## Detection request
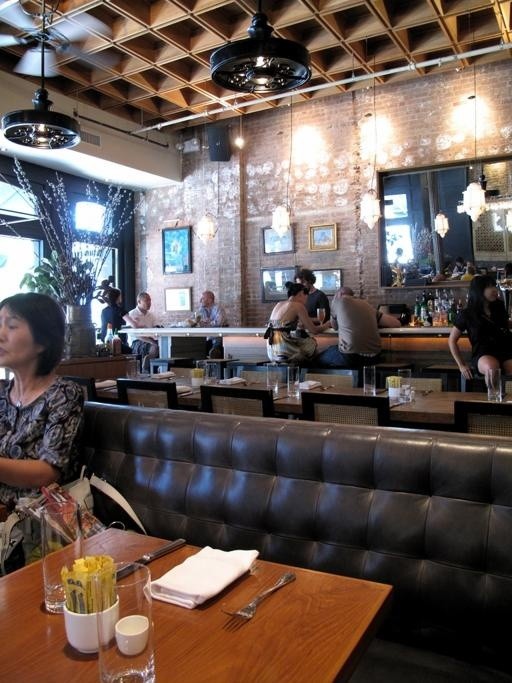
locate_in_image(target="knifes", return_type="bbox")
[116,538,185,584]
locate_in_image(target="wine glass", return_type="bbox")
[317,309,327,326]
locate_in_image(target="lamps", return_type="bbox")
[360,38,383,231]
[2,0,82,150]
[210,0,312,95]
[271,95,293,239]
[435,171,449,238]
[463,14,486,222]
[197,160,220,247]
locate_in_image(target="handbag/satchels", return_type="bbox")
[0,474,147,575]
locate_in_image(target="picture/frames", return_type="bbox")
[162,226,192,274]
[260,266,299,304]
[164,286,192,313]
[263,224,295,255]
[307,222,338,251]
[312,269,342,297]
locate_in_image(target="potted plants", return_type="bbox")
[0,156,147,357]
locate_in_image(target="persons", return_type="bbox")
[183,290,226,359]
[392,248,403,269]
[262,281,330,368]
[99,288,146,355]
[128,292,164,361]
[448,273,511,389]
[452,257,477,281]
[296,269,329,325]
[0,293,84,580]
[320,286,400,370]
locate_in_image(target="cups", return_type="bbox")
[40,501,84,613]
[388,388,400,397]
[267,363,279,397]
[115,614,149,656]
[287,366,300,397]
[93,561,156,682]
[400,369,412,402]
[497,271,506,286]
[205,364,218,384]
[63,595,120,654]
[401,384,411,395]
[363,366,376,396]
[487,368,502,402]
[191,377,203,387]
[126,360,140,380]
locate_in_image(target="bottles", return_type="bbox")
[414,288,464,327]
[96,333,104,357]
[113,328,122,353]
[105,323,114,353]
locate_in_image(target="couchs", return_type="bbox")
[67,400,512,683]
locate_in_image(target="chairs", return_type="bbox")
[378,304,411,329]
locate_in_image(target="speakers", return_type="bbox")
[205,119,235,162]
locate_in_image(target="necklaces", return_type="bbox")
[12,378,36,408]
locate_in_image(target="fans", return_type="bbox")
[0,0,121,76]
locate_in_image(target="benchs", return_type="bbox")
[97,325,229,373]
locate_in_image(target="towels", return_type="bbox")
[147,545,261,610]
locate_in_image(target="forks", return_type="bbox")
[221,571,296,619]
